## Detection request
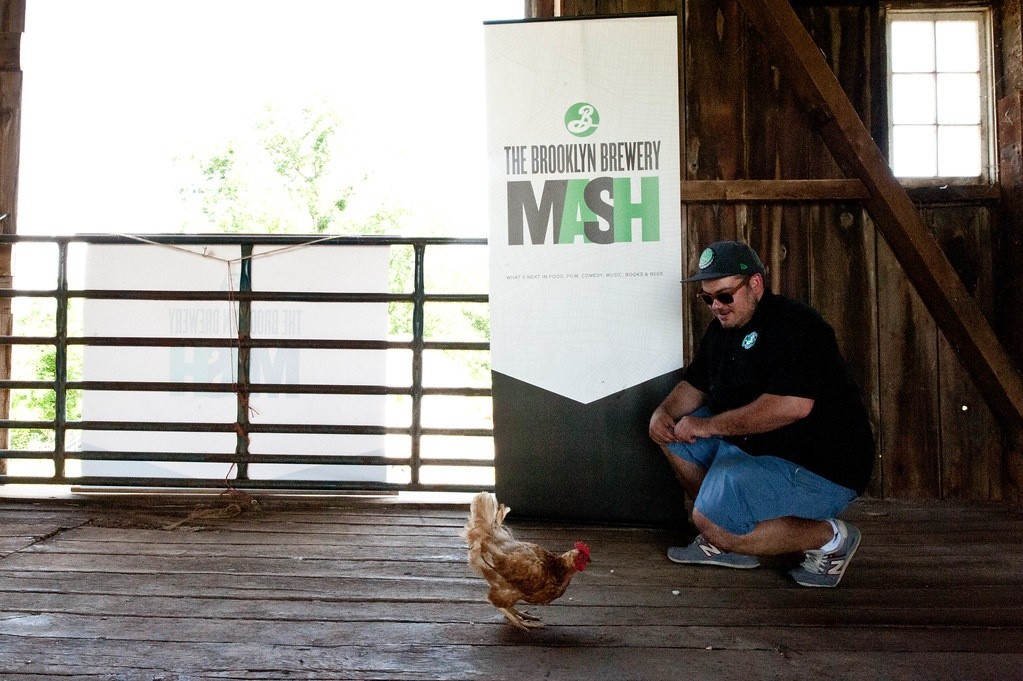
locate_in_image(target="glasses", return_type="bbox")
[697,277,752,306]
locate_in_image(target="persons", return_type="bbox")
[648,238,880,589]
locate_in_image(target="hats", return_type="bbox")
[681,241,766,282]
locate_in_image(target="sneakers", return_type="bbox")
[668,533,762,569]
[789,520,862,585]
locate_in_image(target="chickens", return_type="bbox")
[461,491,592,634]
